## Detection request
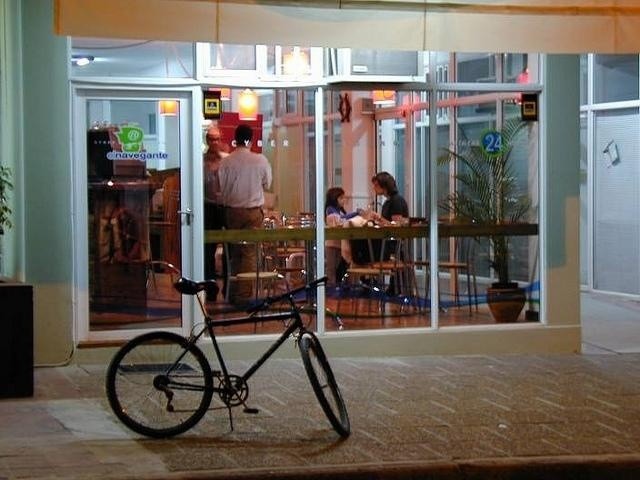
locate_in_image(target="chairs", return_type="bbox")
[222,212,478,328]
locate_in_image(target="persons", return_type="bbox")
[202,127,232,303]
[217,121,274,309]
[324,185,361,294]
[334,171,410,286]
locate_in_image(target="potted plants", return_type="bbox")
[1,163,33,398]
[438,116,532,323]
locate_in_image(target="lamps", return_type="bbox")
[160,101,176,116]
[210,87,231,100]
[372,90,396,104]
[238,89,258,121]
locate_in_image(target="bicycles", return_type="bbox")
[105,276,350,438]
